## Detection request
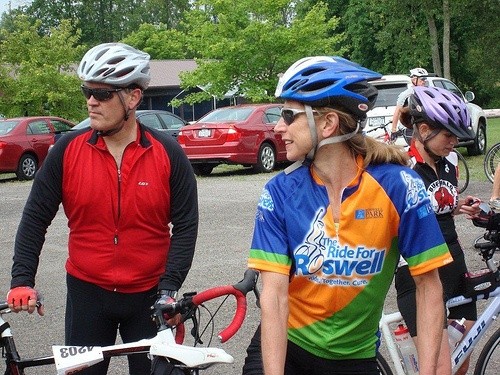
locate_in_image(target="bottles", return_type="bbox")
[447,317,466,353]
[394,324,419,375]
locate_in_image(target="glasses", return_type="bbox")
[80,83,124,102]
[419,78,427,81]
[279,107,321,125]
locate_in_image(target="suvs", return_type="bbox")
[361,74,488,159]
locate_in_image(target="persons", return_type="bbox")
[489,162,500,212]
[6,43,198,375]
[390,68,428,144]
[394,86,482,375]
[243,55,453,375]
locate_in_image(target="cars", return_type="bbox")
[0,116,77,180]
[48,109,191,156]
[177,104,295,177]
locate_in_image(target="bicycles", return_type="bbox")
[0,266,264,375]
[364,119,470,195]
[483,143,500,184]
[372,196,500,375]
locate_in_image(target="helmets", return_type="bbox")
[274,56,383,119]
[76,42,151,91]
[410,68,429,77]
[410,86,475,140]
